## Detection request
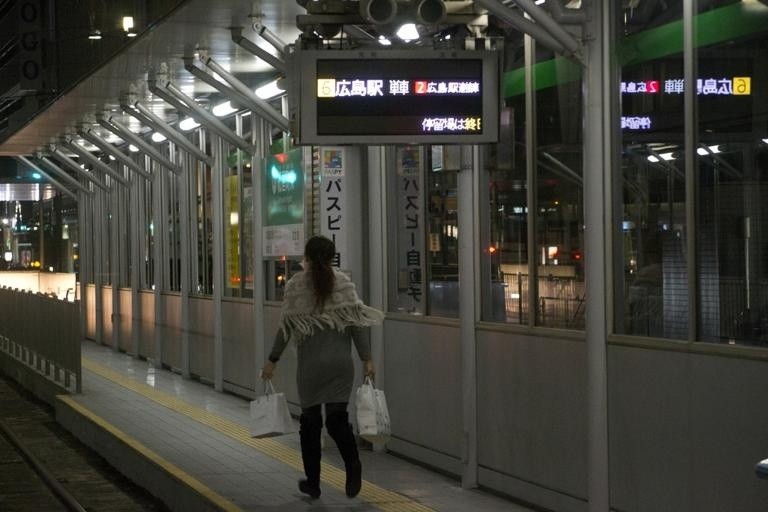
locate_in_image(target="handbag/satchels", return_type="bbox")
[250,377,296,439]
[356,377,392,447]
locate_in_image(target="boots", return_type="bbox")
[298,435,321,498]
[336,433,362,499]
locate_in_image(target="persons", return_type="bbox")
[261,236,375,499]
